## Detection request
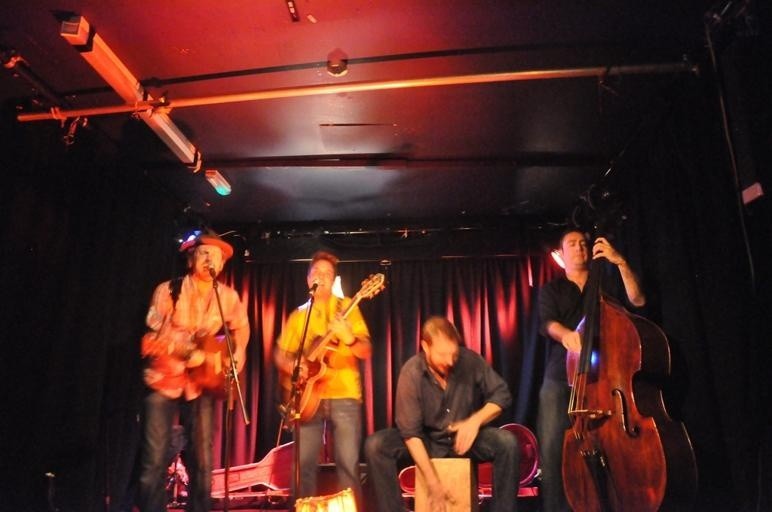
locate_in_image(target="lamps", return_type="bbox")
[60,15,231,198]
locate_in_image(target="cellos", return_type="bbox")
[562,222,699,512]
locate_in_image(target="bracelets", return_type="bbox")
[347,337,358,347]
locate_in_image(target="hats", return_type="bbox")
[178,229,234,260]
[308,250,338,266]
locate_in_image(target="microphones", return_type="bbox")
[308,280,320,294]
[208,264,218,288]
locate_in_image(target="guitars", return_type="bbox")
[141,333,236,398]
[282,273,384,422]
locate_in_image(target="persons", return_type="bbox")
[363,316,521,512]
[273,252,372,512]
[536,228,647,511]
[137,230,249,512]
